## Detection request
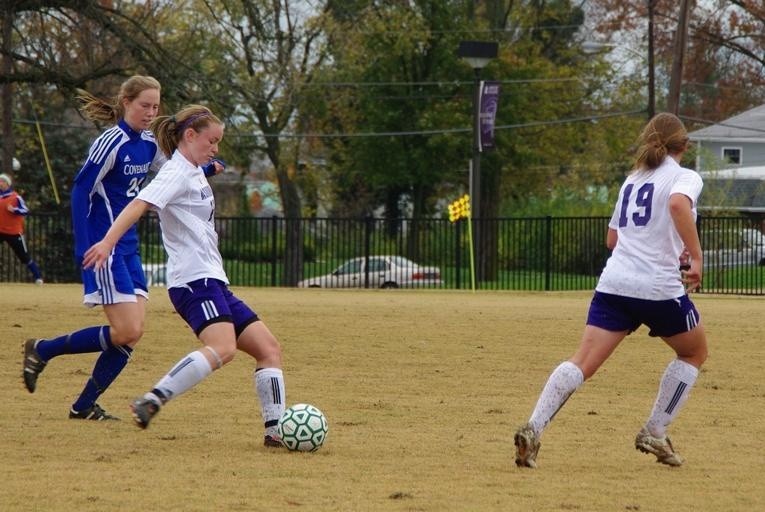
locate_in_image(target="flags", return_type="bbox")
[449,194,472,222]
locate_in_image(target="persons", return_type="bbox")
[516,111,710,469]
[23,75,228,421]
[0,173,44,285]
[83,103,285,446]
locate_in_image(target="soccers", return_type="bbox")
[276,403,328,454]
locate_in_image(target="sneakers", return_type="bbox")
[129,397,159,431]
[513,426,540,471]
[21,337,47,394]
[633,425,684,469]
[34,276,43,285]
[67,404,122,420]
[263,424,283,449]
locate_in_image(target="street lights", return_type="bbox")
[459,41,498,288]
[579,0,655,122]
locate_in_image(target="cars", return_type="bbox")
[297,255,444,289]
[688,228,765,269]
[142,264,167,287]
